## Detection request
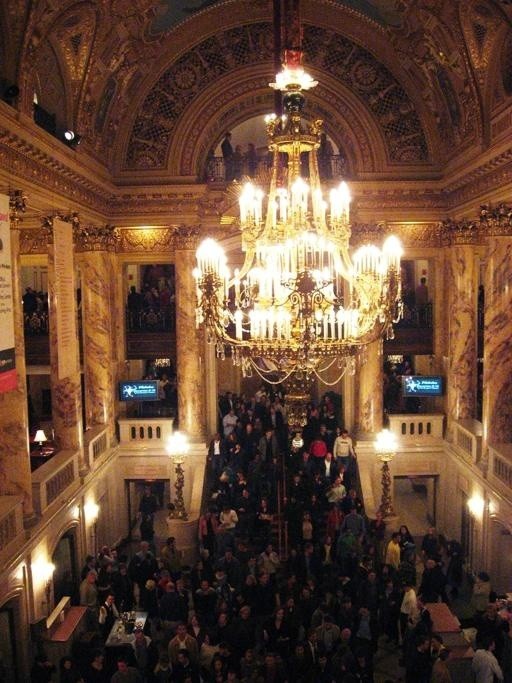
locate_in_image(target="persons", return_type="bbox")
[415,277,429,326]
[221,130,335,182]
[21,285,176,331]
[77,369,512,683]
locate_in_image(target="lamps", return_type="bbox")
[191,0,404,451]
[373,428,397,517]
[166,431,190,520]
[34,430,47,455]
[62,130,82,148]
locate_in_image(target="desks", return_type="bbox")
[425,602,475,683]
[47,607,87,659]
[31,443,55,461]
[104,612,149,648]
